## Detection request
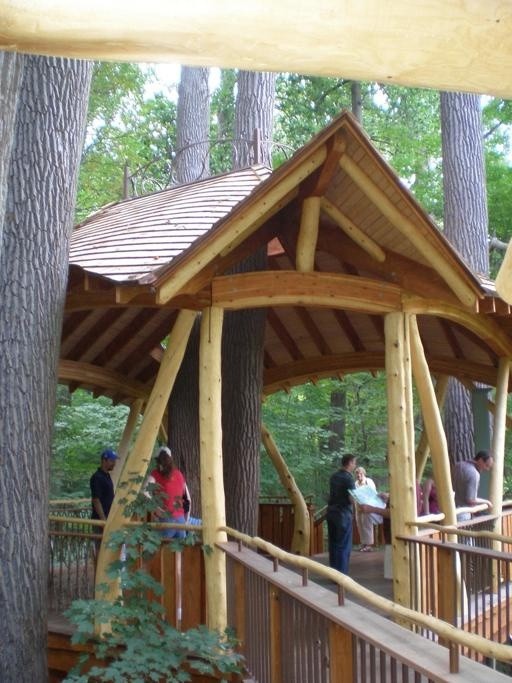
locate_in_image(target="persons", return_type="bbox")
[140,451,187,540]
[425,472,440,513]
[451,448,495,591]
[89,448,122,558]
[152,446,191,535]
[353,465,378,551]
[360,448,423,581]
[325,452,357,583]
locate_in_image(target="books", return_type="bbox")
[348,483,388,524]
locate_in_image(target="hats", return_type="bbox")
[101,450,119,460]
[155,447,171,458]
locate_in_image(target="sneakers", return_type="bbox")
[360,546,372,552]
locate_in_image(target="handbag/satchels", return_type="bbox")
[183,495,190,513]
